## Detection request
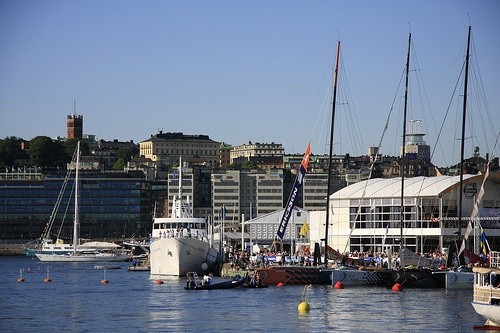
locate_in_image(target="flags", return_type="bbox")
[481,227,487,258]
[299,223,310,237]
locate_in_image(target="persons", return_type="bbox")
[351,249,401,270]
[138,260,140,266]
[230,250,311,266]
[415,251,448,261]
[133,259,136,266]
[186,275,193,283]
[203,274,212,284]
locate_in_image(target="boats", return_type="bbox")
[148,153,218,279]
[183,276,247,291]
[123,239,150,258]
[22,113,136,262]
[472,250,500,324]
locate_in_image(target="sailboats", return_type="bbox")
[428,26,490,289]
[320,33,435,289]
[255,38,362,289]
